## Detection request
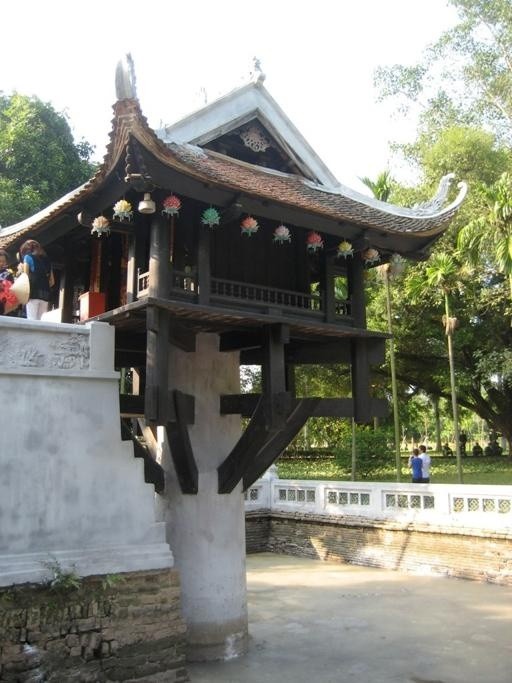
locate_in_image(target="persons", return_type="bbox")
[20,238,56,322]
[441,430,503,457]
[419,445,431,482]
[408,449,422,482]
[0,250,14,314]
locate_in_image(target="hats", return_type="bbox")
[9,272,32,306]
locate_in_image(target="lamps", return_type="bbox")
[138,193,155,214]
